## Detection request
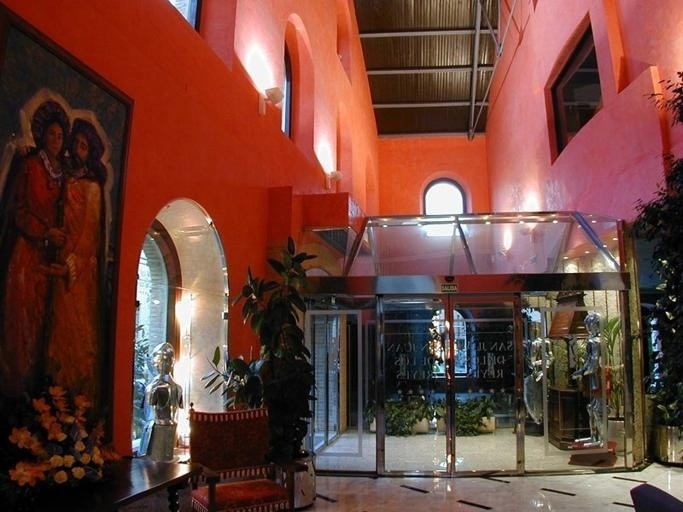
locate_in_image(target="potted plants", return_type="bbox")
[362,394,432,438]
[602,315,627,451]
[638,154,682,468]
[199,236,348,512]
[432,397,497,437]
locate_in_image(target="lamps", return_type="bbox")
[257,86,283,116]
[325,170,343,189]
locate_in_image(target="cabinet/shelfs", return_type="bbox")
[547,384,591,451]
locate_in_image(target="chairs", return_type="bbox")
[190,404,307,511]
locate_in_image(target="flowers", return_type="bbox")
[5,384,107,489]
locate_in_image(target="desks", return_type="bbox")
[0,456,201,512]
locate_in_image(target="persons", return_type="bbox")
[40,118,108,395]
[0,114,67,403]
[145,342,184,426]
[570,313,608,447]
